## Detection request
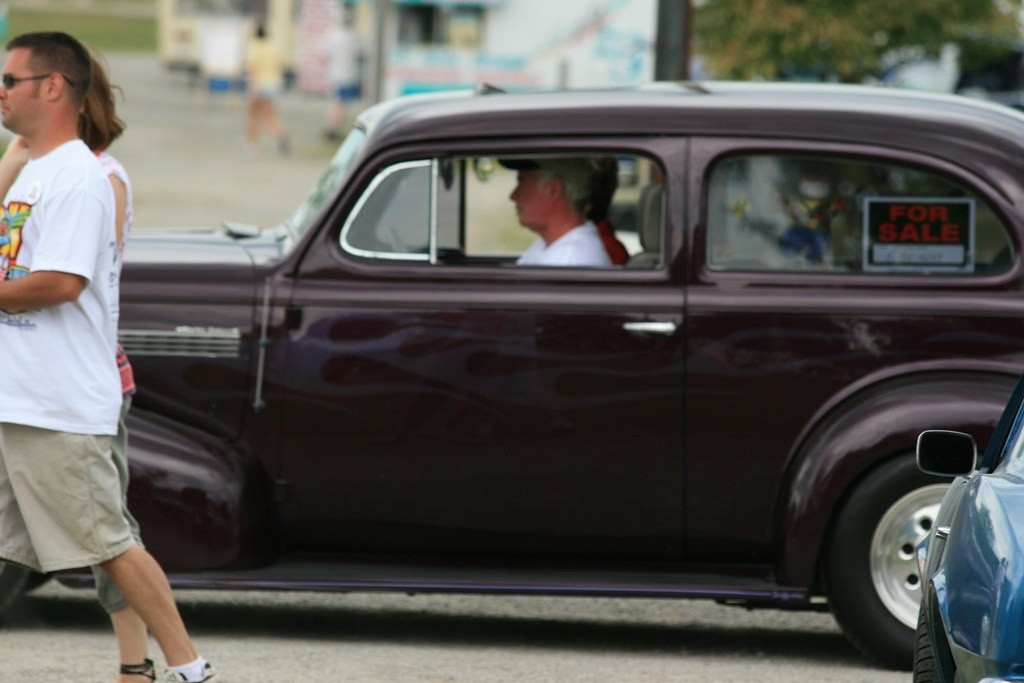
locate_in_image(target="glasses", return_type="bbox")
[3,75,73,89]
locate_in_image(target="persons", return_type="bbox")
[246,11,371,153]
[0,30,214,683]
[499,157,633,271]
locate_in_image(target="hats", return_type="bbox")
[498,159,600,192]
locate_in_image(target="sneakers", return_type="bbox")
[158,659,217,683]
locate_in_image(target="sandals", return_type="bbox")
[120,659,156,683]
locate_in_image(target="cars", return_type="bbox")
[0,81,1024,674]
[915,372,1024,683]
